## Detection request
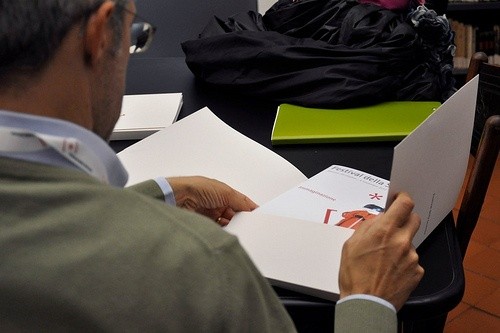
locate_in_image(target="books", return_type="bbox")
[109,70,482,301]
[270,97,442,149]
[107,92,184,141]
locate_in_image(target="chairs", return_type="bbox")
[402,51,500,333]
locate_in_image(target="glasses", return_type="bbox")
[115,3,158,55]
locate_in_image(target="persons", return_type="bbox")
[0,0,424,333]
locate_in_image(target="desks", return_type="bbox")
[111,0,466,333]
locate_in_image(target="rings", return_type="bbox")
[216,217,222,223]
